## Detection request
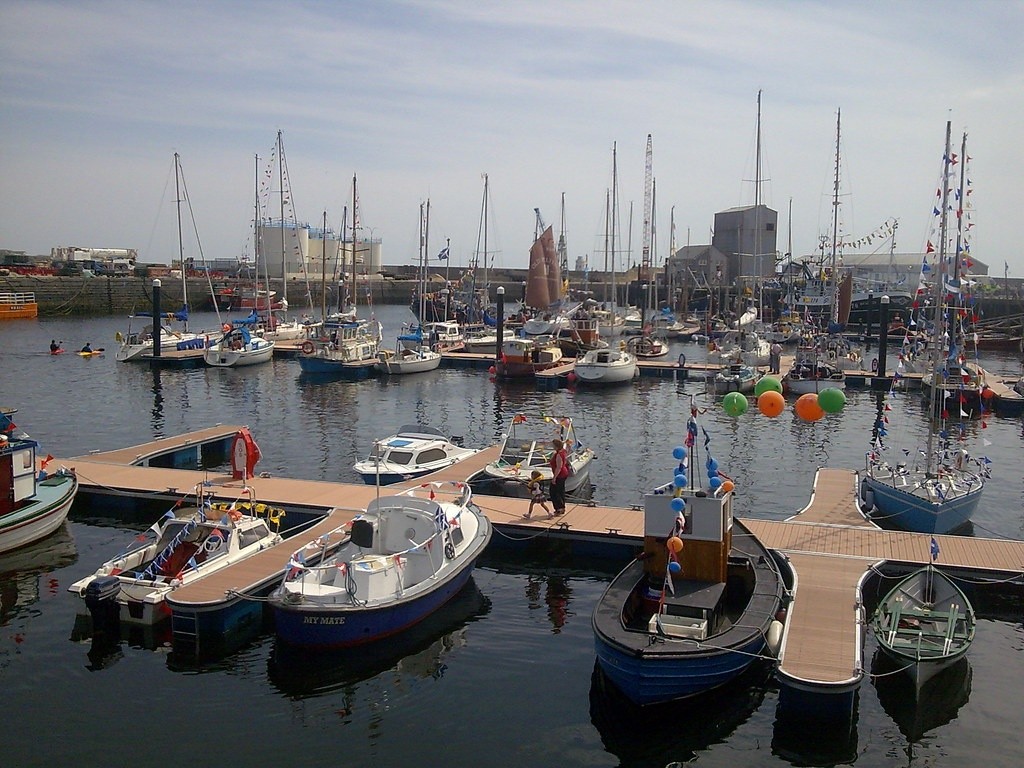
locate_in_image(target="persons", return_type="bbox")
[548,439,569,515]
[81,343,92,352]
[523,470,554,520]
[231,338,243,351]
[769,339,783,374]
[50,340,60,351]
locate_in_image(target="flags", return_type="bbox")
[931,535,940,559]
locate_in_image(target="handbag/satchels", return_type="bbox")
[566,460,578,476]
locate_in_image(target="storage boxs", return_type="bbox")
[649,613,708,640]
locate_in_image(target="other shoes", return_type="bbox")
[554,508,565,514]
[768,370,772,371]
[547,514,553,520]
[523,513,530,518]
[777,372,779,374]
[772,372,775,374]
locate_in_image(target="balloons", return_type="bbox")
[666,376,846,572]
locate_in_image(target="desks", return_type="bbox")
[663,579,726,636]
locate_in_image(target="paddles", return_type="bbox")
[58,341,62,348]
[75,348,105,352]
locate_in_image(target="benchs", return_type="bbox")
[882,627,968,639]
[885,607,967,620]
[891,643,960,651]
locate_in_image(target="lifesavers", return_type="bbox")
[850,353,856,362]
[872,358,878,372]
[302,340,314,354]
[0,434,8,447]
[679,353,686,366]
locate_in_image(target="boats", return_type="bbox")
[574,348,638,383]
[51,349,64,355]
[76,351,102,355]
[0,406,80,555]
[785,359,847,396]
[484,412,597,501]
[66,503,285,627]
[864,461,983,534]
[713,363,763,394]
[352,424,480,486]
[378,346,442,374]
[494,339,563,375]
[920,371,989,416]
[114,284,384,378]
[266,480,494,651]
[409,274,966,374]
[590,478,785,709]
[873,562,977,704]
